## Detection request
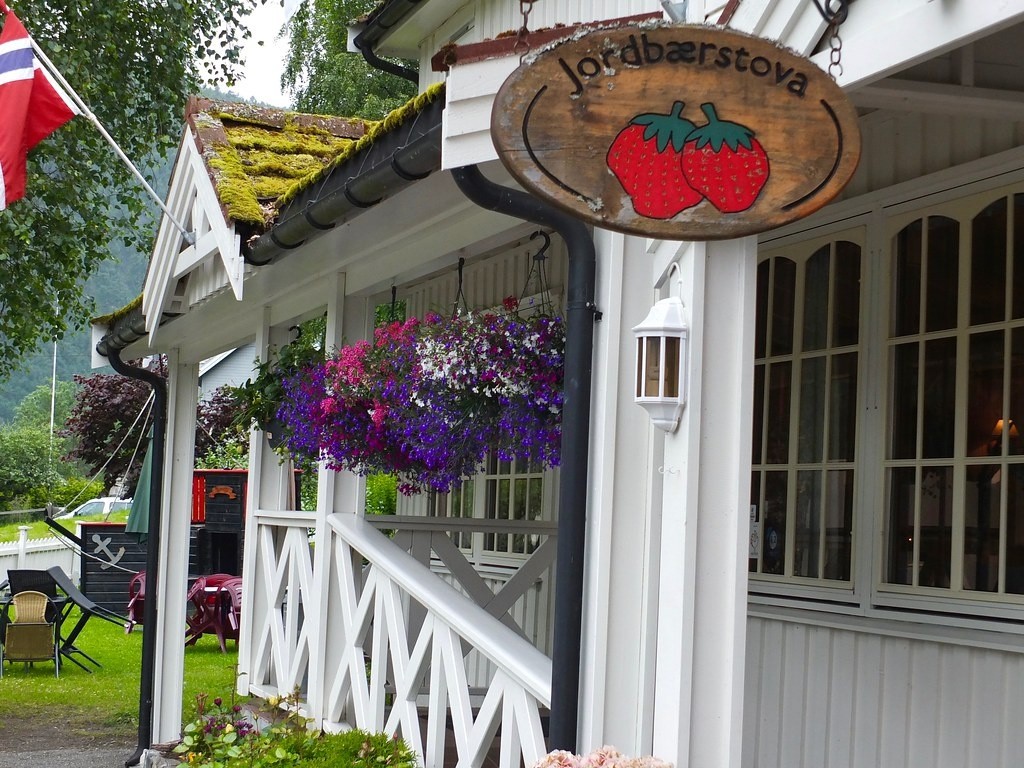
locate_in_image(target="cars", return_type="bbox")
[53,497,135,522]
[186,574,227,628]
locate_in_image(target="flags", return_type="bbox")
[1,0,79,213]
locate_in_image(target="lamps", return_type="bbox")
[993,420,1020,439]
[631,298,689,433]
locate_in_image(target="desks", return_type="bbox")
[0,597,68,605]
[201,587,242,649]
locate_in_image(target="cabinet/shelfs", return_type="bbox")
[78,521,204,616]
[192,468,303,532]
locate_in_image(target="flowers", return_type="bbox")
[232,296,570,496]
[529,744,675,768]
[172,663,422,768]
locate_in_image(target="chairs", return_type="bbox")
[126,571,146,633]
[7,570,63,597]
[0,591,61,679]
[47,566,137,674]
[215,578,242,652]
[185,577,213,647]
[191,574,239,647]
